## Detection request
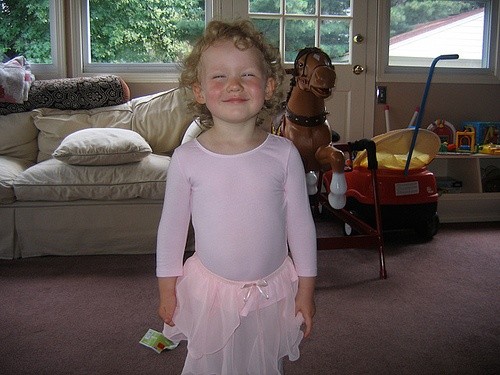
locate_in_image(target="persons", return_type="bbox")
[154,15,319,375]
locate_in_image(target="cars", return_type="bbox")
[319,127,442,237]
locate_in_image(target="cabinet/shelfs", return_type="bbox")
[427,152,500,223]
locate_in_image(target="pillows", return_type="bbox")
[52,127,153,166]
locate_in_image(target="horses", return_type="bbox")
[270,45,348,209]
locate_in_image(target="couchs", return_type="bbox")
[1,74,215,266]
